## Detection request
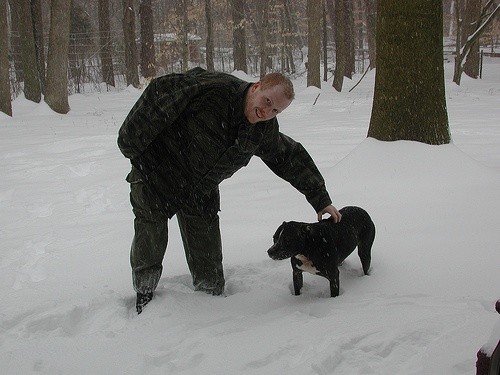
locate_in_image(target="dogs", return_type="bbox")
[267,206,376,298]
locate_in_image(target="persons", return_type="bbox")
[118,65,342,314]
